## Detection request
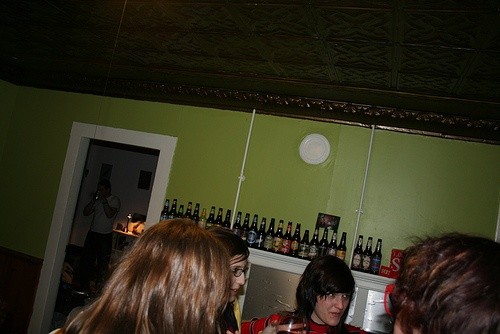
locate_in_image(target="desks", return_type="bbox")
[112,228,140,250]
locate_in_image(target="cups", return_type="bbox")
[271,310,309,334]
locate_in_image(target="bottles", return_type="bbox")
[160,199,382,275]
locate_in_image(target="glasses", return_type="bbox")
[231,267,249,277]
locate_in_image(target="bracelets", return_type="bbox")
[103,201,108,204]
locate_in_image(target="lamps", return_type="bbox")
[126,213,132,232]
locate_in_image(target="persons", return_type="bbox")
[78,179,121,285]
[394,232,500,334]
[206,227,371,334]
[52,218,231,334]
